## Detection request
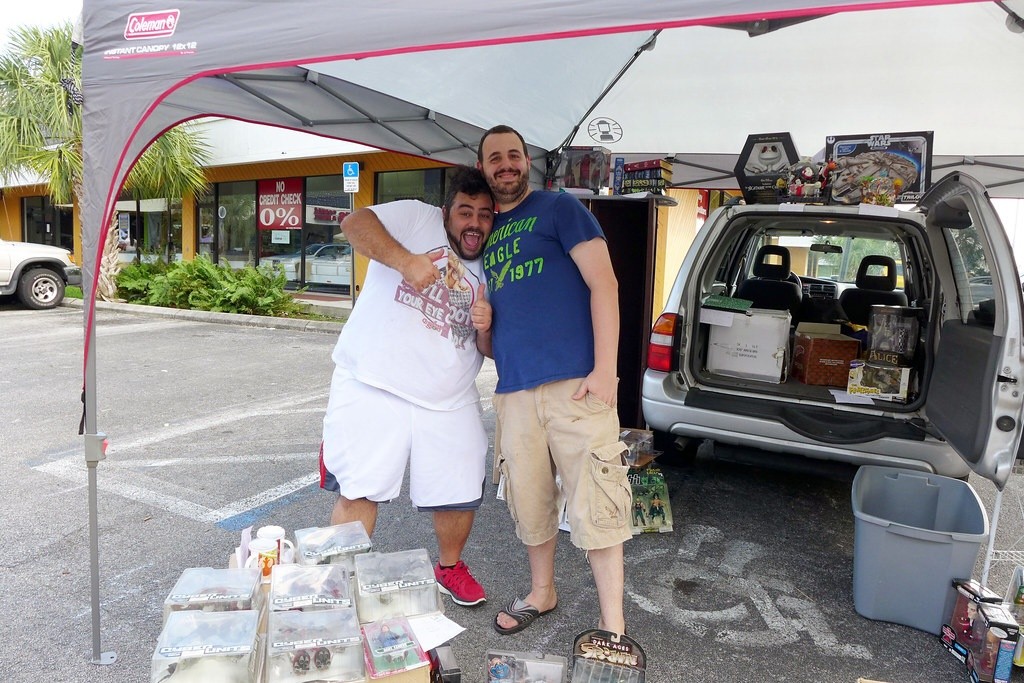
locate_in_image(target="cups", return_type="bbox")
[244,539,278,583]
[257,525,294,565]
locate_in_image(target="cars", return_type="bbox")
[0,237,83,310]
[258,245,349,294]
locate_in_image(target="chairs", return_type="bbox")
[839,255,908,326]
[733,245,802,324]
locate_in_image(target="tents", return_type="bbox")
[79,0,1024,665]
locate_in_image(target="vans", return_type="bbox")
[641,168,1024,492]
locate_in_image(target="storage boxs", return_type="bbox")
[847,359,919,404]
[700,307,793,384]
[866,305,924,367]
[484,629,647,683]
[791,321,862,388]
[149,521,467,683]
[851,466,989,637]
[938,578,1020,683]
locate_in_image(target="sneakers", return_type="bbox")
[433,560,486,605]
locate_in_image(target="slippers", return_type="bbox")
[493,596,558,634]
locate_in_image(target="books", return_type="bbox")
[614,157,673,195]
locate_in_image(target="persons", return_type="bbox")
[319,123,634,642]
